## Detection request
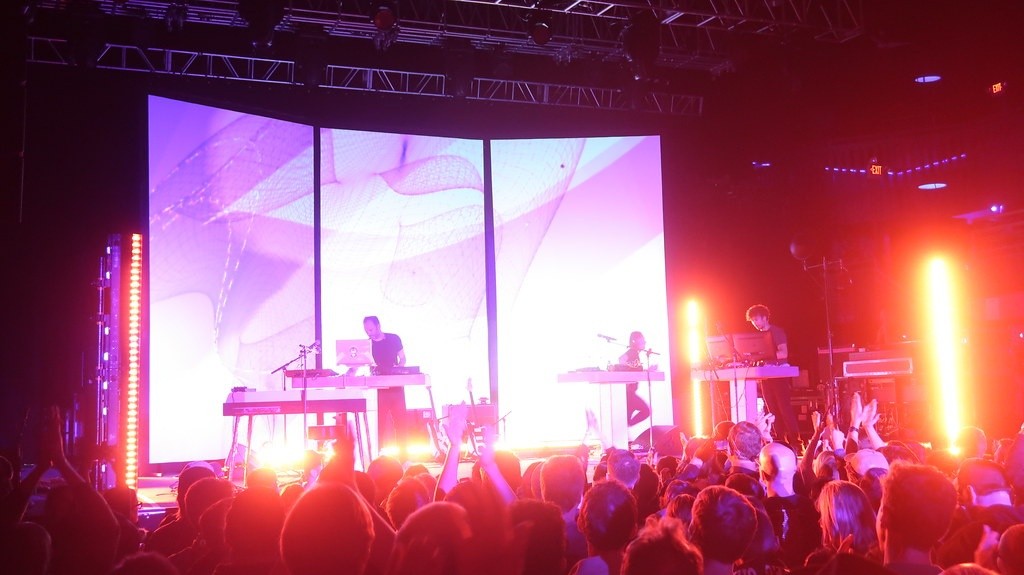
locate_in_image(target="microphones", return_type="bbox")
[598,334,616,341]
[308,341,320,349]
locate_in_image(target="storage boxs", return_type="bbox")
[842,358,914,377]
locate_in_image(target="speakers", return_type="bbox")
[634,425,682,456]
[406,409,438,442]
[442,404,499,445]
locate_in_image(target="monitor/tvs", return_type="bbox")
[707,332,777,363]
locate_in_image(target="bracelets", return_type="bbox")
[849,427,860,432]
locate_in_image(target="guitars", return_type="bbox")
[419,384,457,466]
[462,376,496,461]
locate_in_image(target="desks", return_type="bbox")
[691,367,799,426]
[292,374,424,436]
[557,372,665,450]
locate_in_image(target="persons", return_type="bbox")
[618,332,650,426]
[746,305,803,457]
[363,316,412,467]
[0,394,1024,575]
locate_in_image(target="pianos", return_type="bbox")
[223,386,367,416]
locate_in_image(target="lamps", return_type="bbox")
[521,6,553,45]
[369,0,401,30]
[621,10,661,82]
[238,0,286,49]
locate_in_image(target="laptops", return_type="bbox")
[336,339,375,365]
